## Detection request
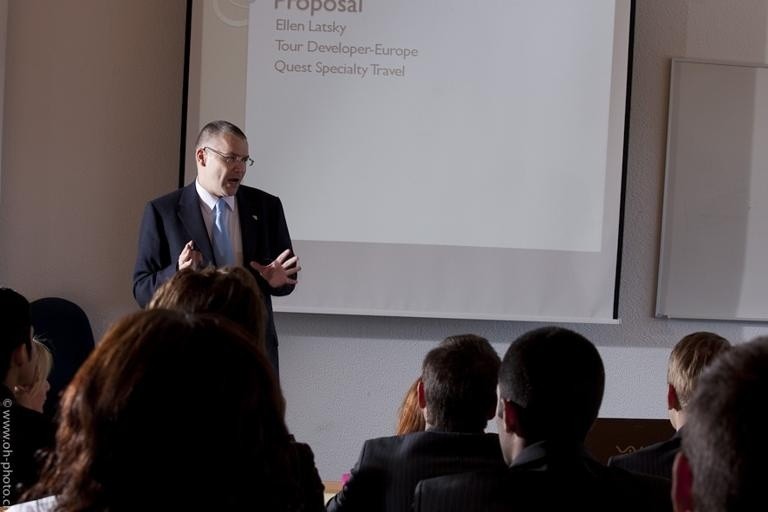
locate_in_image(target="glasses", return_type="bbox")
[203,146,254,167]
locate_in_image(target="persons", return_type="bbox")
[0,264,767,512]
[133,119,302,372]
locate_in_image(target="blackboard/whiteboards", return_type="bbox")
[650,56,767,323]
